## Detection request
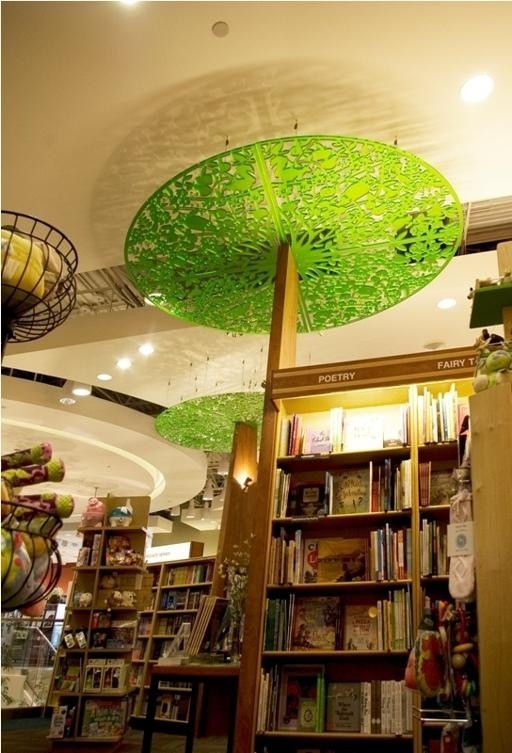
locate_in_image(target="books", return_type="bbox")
[279,382,470,456]
[257,664,415,734]
[268,519,451,585]
[273,457,430,519]
[262,587,450,652]
[130,561,219,721]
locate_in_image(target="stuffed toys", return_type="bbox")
[80,497,106,527]
[110,498,133,527]
[84,663,125,695]
[105,590,136,612]
[1,439,75,616]
